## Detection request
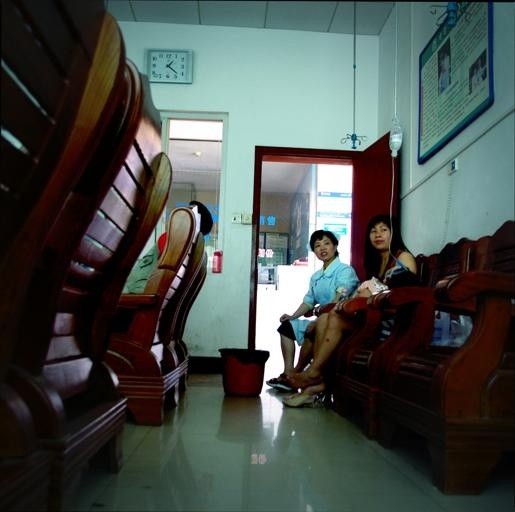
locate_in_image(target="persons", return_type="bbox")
[267,227,360,390]
[122,198,214,294]
[280,214,417,408]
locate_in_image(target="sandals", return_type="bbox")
[266,372,295,391]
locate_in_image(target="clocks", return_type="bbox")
[144,48,193,84]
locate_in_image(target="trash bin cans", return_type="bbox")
[218,348,270,396]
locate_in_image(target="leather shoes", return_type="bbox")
[282,391,319,407]
